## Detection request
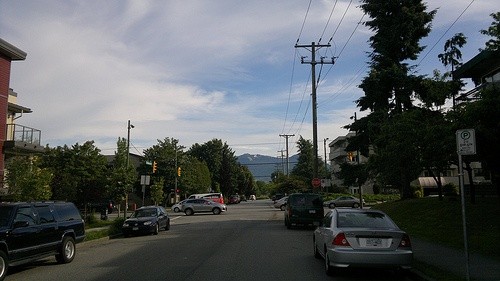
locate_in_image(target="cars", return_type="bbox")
[171,200,185,213]
[249,195,256,200]
[121,205,170,238]
[274,196,289,211]
[227,194,247,205]
[322,195,365,209]
[312,209,414,279]
[181,198,228,216]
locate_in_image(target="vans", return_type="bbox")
[187,193,224,206]
[284,192,324,228]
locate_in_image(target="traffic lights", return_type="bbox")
[152,161,157,173]
[177,167,181,176]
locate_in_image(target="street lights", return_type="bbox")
[324,138,329,200]
[123,119,134,220]
[350,111,362,208]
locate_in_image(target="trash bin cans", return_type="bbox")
[101,207,108,220]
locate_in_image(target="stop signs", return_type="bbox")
[311,178,320,187]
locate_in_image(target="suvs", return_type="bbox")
[0,200,87,281]
